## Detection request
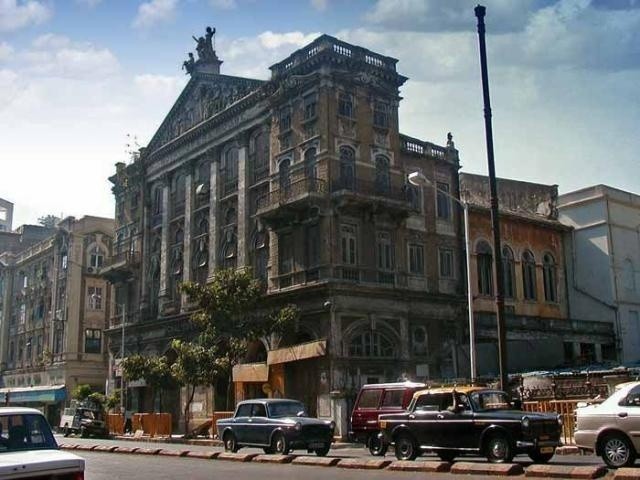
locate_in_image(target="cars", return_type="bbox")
[216,398,336,456]
[0,406,85,480]
[574,381,639,470]
[349,383,562,464]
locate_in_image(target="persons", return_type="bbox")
[593,387,609,404]
[206,25,217,51]
[120,405,134,436]
[192,34,206,58]
[183,52,195,76]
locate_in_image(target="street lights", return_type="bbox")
[92,294,126,410]
[407,172,477,384]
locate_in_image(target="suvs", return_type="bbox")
[60,407,107,438]
